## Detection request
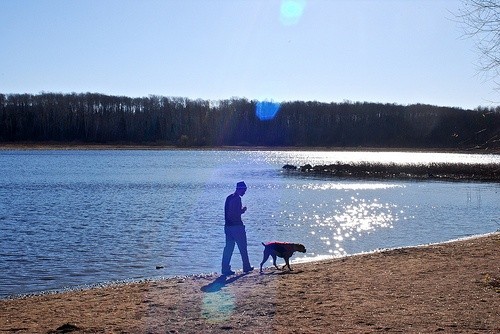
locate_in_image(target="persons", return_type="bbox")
[220,181,255,276]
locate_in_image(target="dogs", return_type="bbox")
[259,241,307,273]
